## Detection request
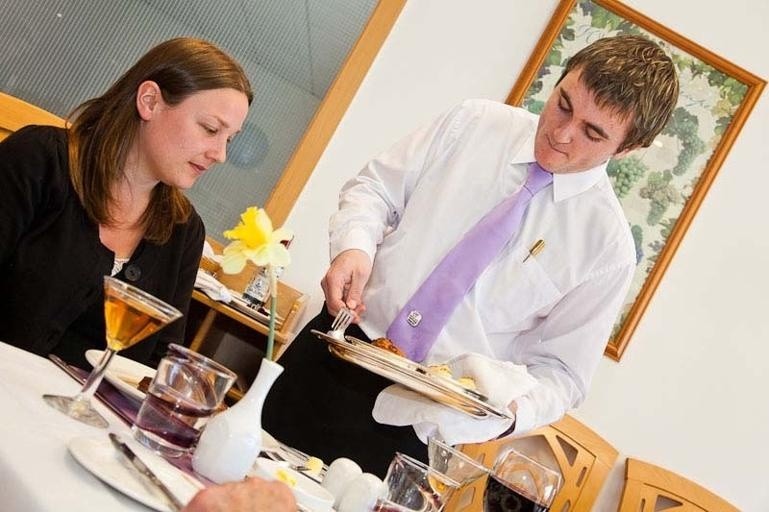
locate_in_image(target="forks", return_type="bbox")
[331,305,354,333]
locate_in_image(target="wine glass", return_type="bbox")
[42,273,184,430]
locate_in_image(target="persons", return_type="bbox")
[261,35,679,511]
[0,36,255,374]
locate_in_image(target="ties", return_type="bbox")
[385,160,554,363]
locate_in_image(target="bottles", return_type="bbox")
[279,291,309,334]
[238,230,296,312]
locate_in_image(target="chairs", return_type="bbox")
[438,409,621,510]
[613,449,739,511]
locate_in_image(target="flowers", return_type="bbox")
[219,206,300,362]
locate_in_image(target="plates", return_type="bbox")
[261,432,328,481]
[67,424,200,510]
[253,455,334,511]
[346,336,487,402]
[198,271,283,329]
[87,346,157,407]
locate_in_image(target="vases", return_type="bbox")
[192,355,284,486]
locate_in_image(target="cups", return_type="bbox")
[135,341,237,460]
[322,435,563,511]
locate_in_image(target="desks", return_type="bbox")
[191,285,297,368]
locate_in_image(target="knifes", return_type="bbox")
[108,431,185,511]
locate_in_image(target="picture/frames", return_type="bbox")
[503,0,768,364]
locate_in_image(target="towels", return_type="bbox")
[372,352,539,451]
[194,269,233,306]
